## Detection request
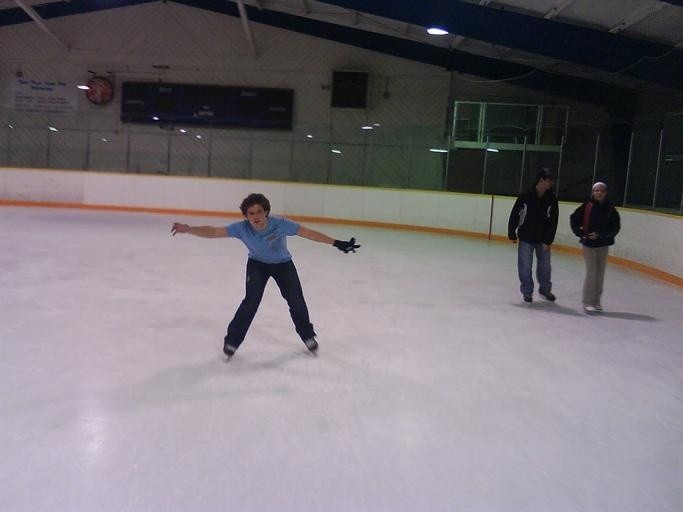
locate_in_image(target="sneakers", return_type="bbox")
[540,291,555,301]
[303,337,318,351]
[224,343,236,354]
[524,294,532,302]
[584,305,602,312]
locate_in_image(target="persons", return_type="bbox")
[171,192,360,357]
[570,182,620,315]
[505,167,558,302]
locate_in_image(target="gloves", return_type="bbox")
[333,237,360,254]
[588,232,599,241]
